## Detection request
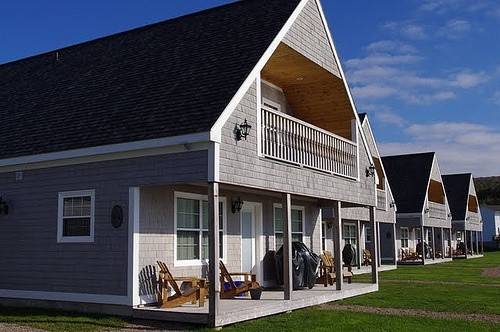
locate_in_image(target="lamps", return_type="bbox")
[390,201,395,209]
[366,164,375,177]
[425,209,429,213]
[234,118,252,141]
[327,217,335,232]
[231,196,244,214]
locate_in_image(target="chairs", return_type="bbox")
[157,261,209,308]
[401,249,419,261]
[363,250,372,266]
[220,260,259,299]
[316,252,353,287]
